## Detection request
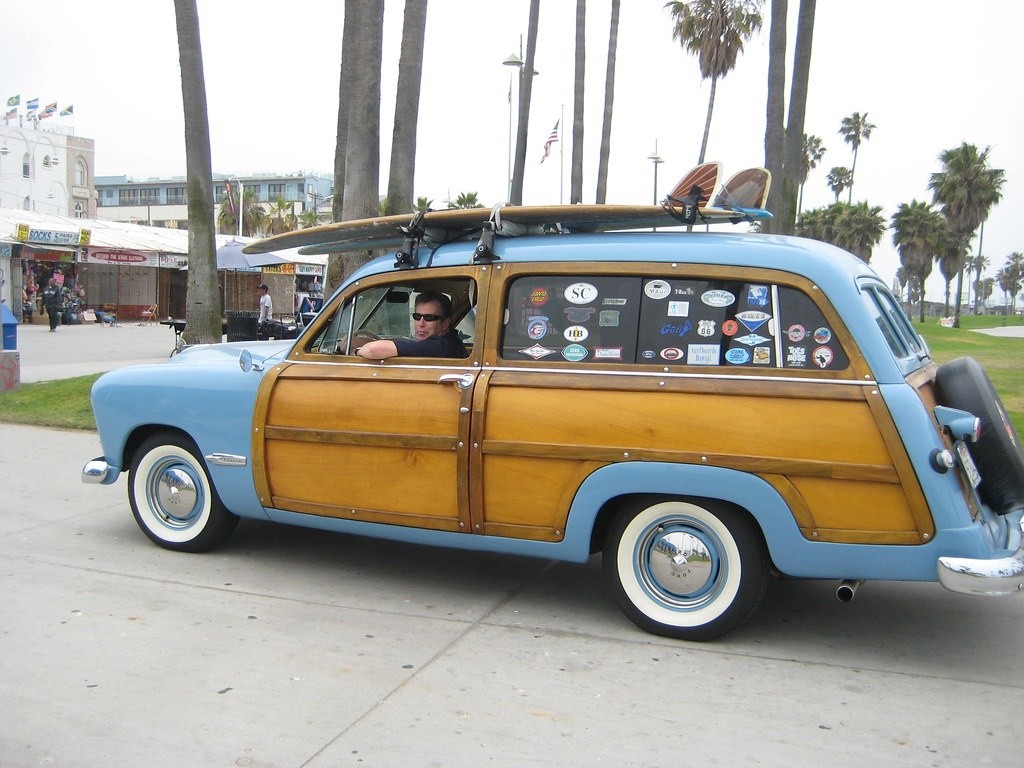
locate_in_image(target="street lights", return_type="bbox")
[1,130,62,210]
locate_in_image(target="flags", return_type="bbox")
[60,105,73,117]
[26,98,39,109]
[7,95,20,106]
[38,101,57,120]
[2,108,17,120]
[27,110,37,121]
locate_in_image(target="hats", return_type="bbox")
[256,284,268,291]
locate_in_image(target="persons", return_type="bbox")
[968,300,976,316]
[257,283,273,340]
[40,278,63,332]
[353,291,468,360]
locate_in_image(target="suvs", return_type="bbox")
[83,161,1024,644]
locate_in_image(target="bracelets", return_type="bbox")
[354,348,359,356]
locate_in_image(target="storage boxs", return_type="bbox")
[81,312,97,324]
[31,297,50,325]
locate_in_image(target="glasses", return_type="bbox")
[413,313,444,322]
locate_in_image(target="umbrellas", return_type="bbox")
[179,238,294,311]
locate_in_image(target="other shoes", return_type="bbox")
[49,328,55,331]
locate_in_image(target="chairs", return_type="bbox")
[102,303,117,328]
[139,304,157,327]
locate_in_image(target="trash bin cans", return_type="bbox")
[225,310,261,342]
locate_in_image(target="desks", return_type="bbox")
[160,320,227,358]
